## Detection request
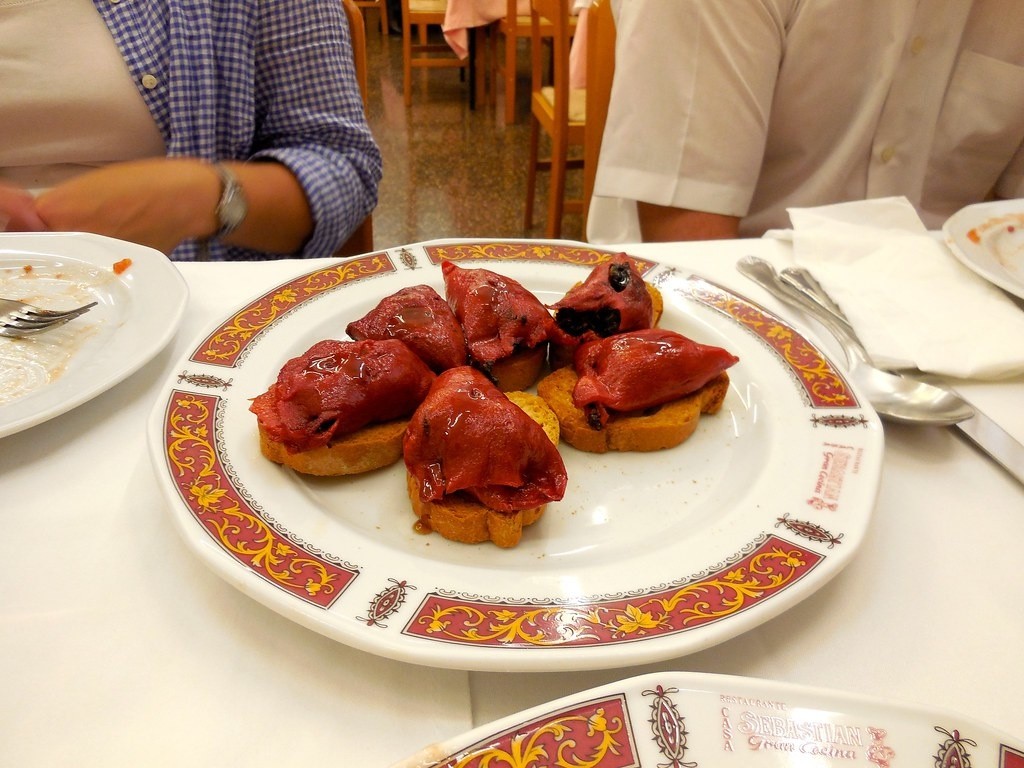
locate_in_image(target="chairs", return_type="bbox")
[330,0,618,256]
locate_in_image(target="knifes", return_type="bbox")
[778,266,1024,483]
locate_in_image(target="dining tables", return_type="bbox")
[0,230,1023,768]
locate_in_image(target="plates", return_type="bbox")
[941,199,1024,301]
[1,231,190,443]
[148,239,884,673]
[384,671,1023,768]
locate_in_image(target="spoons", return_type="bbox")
[736,255,975,423]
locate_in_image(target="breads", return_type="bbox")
[407,392,562,548]
[551,280,664,329]
[257,417,412,476]
[537,363,730,452]
[476,339,548,392]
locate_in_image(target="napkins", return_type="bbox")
[763,193,1023,382]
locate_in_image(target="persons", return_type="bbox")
[0,1,380,263]
[585,0,1023,245]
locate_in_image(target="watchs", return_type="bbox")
[196,160,247,244]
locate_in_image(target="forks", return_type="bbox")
[1,297,98,337]
